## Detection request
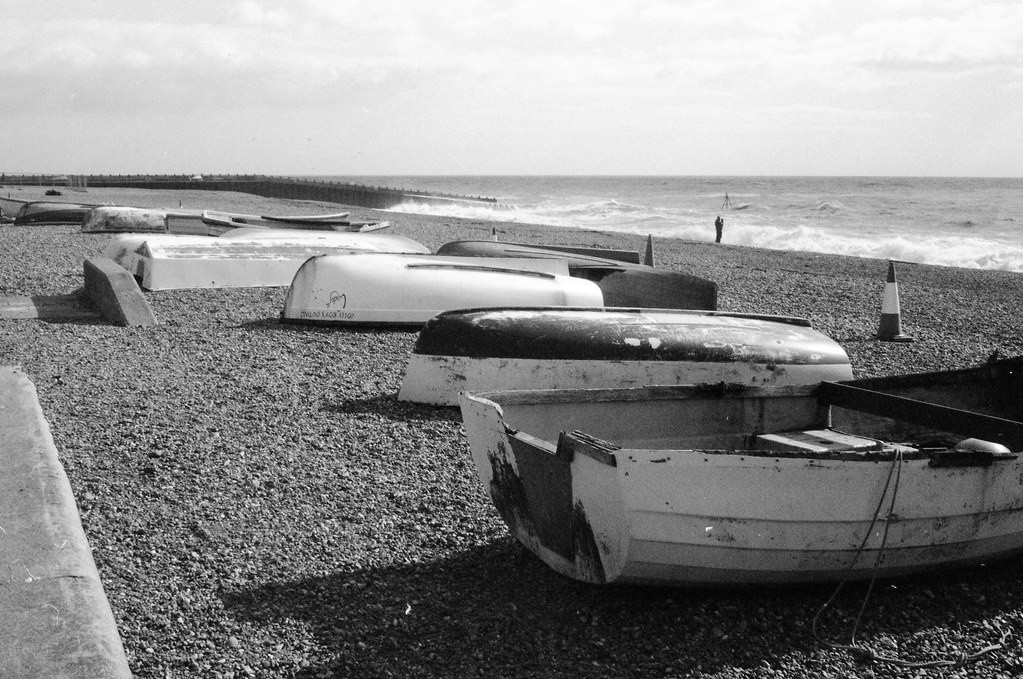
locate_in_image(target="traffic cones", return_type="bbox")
[869,258,918,344]
[642,234,655,268]
[491,226,498,242]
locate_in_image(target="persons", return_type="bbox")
[714,216,723,243]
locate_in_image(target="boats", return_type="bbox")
[434,240,720,312]
[1,195,393,238]
[101,223,433,291]
[278,252,608,326]
[457,348,1023,591]
[396,304,857,410]
